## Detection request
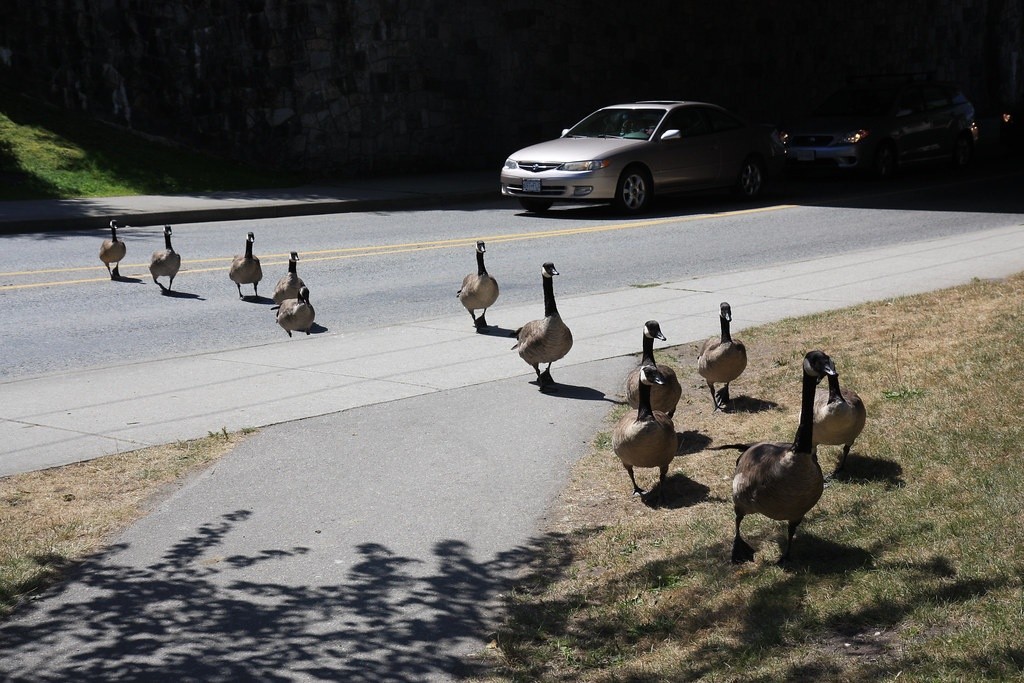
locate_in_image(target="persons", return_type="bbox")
[619,113,637,130]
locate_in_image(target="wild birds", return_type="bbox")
[624,320,683,418]
[512,262,574,384]
[98,220,126,278]
[150,224,181,292]
[229,231,262,302]
[272,251,315,337]
[730,350,868,566]
[699,302,748,412]
[456,239,499,334]
[611,366,679,495]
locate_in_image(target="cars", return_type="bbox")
[998,99,1024,153]
[773,83,980,190]
[498,100,774,217]
